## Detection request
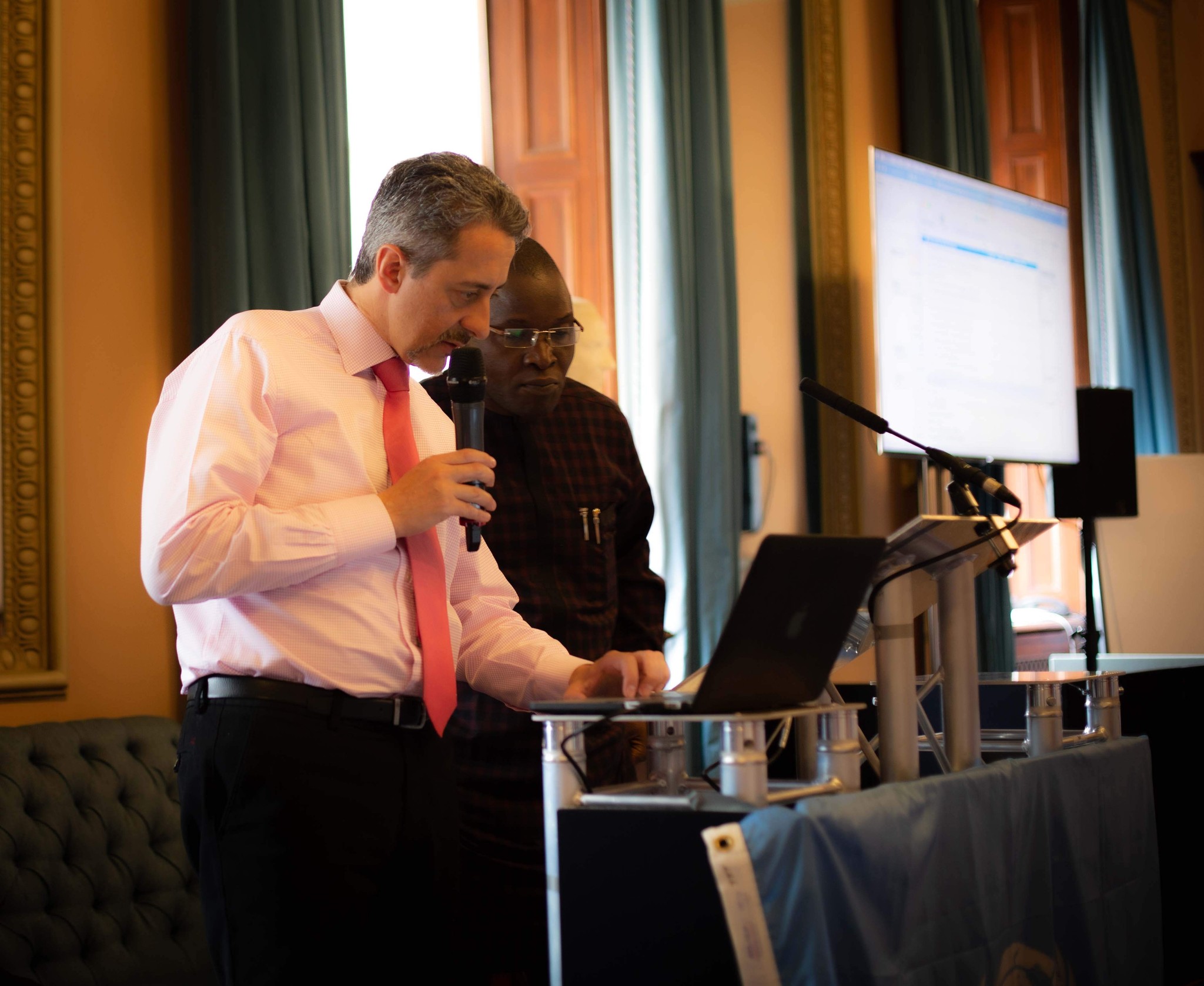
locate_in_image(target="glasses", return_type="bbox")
[489,317,584,349]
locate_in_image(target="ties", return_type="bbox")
[371,357,458,737]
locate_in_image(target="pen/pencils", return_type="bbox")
[592,508,603,546]
[579,508,594,541]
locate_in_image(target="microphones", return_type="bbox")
[447,346,487,552]
[798,376,1023,509]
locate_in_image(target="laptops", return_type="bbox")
[528,533,890,717]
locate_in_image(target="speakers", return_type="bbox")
[741,414,761,529]
[1052,387,1138,519]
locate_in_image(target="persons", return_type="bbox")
[137,153,673,986]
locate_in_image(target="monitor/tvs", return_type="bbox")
[866,144,1080,465]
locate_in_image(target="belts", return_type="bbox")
[187,675,431,730]
